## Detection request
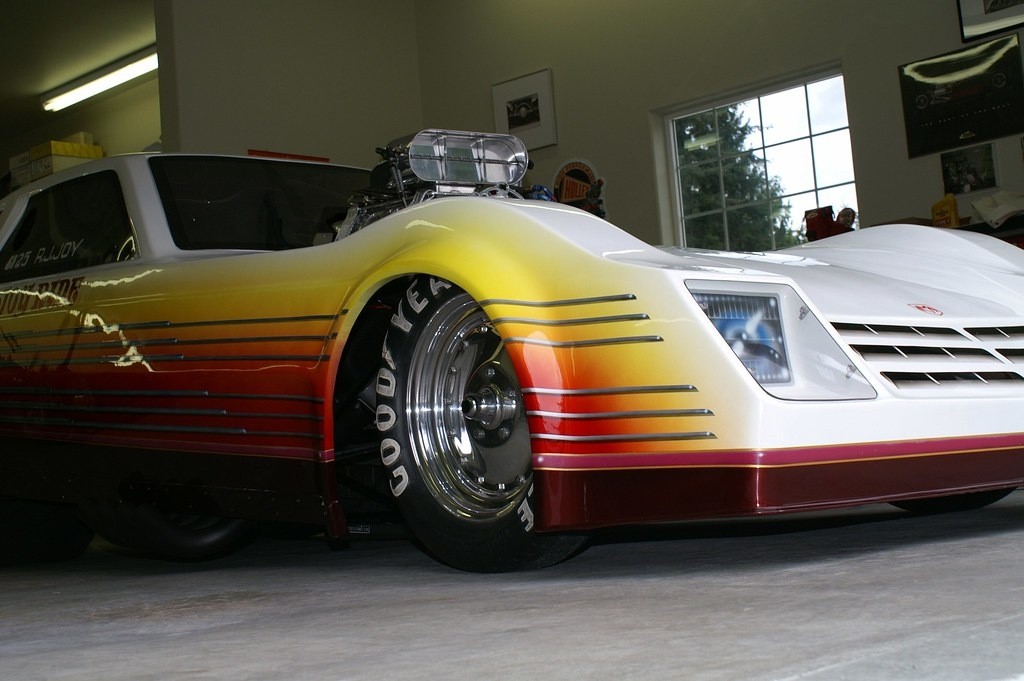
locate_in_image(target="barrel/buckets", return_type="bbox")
[931,193,960,228]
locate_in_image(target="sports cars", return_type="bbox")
[0,127,1024,577]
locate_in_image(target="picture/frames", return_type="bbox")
[954,0,1024,45]
[492,68,557,151]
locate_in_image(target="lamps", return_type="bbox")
[39,45,158,112]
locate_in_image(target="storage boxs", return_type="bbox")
[31,140,103,183]
[9,152,28,186]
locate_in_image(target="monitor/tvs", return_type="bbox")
[898,32,1024,160]
